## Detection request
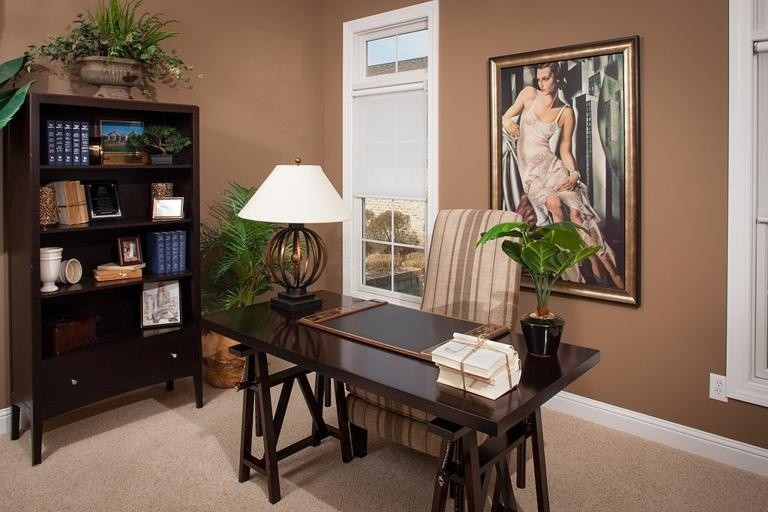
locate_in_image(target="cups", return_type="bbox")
[55,257,83,285]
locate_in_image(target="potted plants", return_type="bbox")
[125,125,192,164]
[24,0,206,103]
[192,179,316,391]
[471,210,604,361]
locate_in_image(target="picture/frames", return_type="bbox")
[81,178,124,221]
[138,280,182,331]
[486,33,646,311]
[115,235,142,266]
[151,196,184,220]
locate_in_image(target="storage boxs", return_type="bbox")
[47,308,98,355]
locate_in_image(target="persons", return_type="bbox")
[499,59,627,295]
[151,281,175,322]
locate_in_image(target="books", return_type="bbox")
[429,330,520,403]
[32,101,191,282]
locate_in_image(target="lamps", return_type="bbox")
[237,156,355,319]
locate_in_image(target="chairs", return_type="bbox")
[341,204,530,500]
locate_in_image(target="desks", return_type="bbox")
[198,286,605,512]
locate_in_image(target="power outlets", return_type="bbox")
[707,372,727,403]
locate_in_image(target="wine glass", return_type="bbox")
[38,247,64,293]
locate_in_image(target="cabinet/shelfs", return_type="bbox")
[5,93,206,468]
